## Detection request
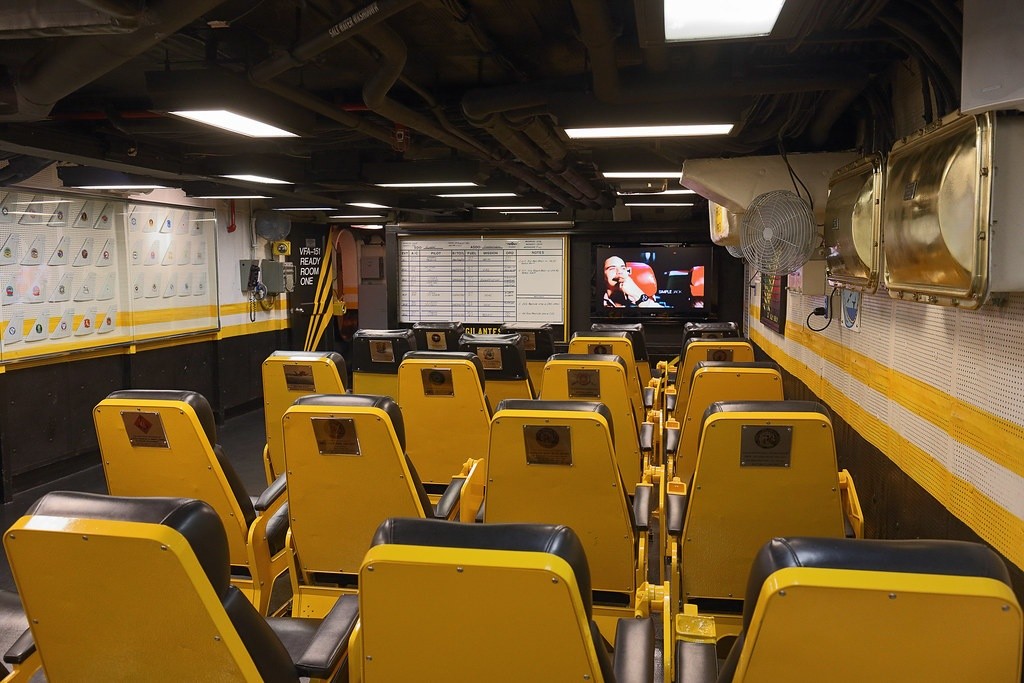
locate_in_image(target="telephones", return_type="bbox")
[248,264,260,288]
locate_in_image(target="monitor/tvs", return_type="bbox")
[590,241,720,322]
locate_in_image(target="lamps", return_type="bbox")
[57,165,179,190]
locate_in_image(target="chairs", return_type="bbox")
[459,333,536,416]
[664,337,755,421]
[2,491,359,683]
[541,354,653,496]
[592,323,665,387]
[568,332,655,429]
[353,328,417,402]
[261,349,354,487]
[282,393,466,620]
[348,516,655,683]
[0,590,49,683]
[412,321,466,352]
[501,321,555,396]
[661,361,785,555]
[667,322,740,382]
[666,398,865,616]
[474,399,653,620]
[397,350,492,486]
[674,535,1024,683]
[93,389,295,618]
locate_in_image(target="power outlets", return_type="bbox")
[824,295,840,319]
[754,281,761,296]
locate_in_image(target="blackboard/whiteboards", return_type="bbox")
[398,237,565,325]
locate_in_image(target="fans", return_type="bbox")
[739,191,826,276]
[725,245,750,265]
[249,207,291,248]
